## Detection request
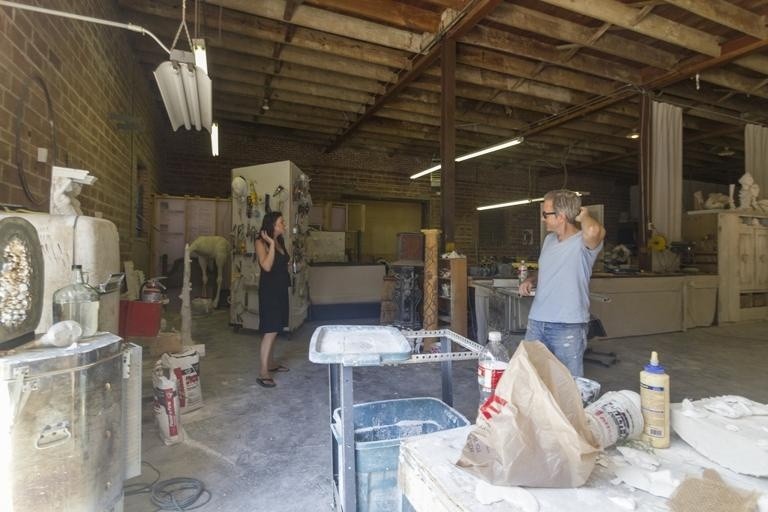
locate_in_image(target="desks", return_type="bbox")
[467,273,720,347]
[395,399,768,511]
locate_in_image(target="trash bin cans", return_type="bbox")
[331,397,470,512]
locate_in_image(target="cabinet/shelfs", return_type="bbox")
[693,251,718,265]
[738,223,767,292]
[438,258,468,338]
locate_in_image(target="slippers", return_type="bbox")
[268,365,290,373]
[256,376,276,388]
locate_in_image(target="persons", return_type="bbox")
[518,187,607,386]
[253,211,292,387]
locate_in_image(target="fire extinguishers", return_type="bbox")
[139,277,168,302]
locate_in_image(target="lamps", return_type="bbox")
[408,134,526,180]
[472,163,591,212]
[151,0,221,159]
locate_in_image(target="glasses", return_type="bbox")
[541,210,556,219]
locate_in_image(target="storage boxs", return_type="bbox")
[121,332,184,358]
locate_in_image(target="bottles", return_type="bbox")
[517,258,527,286]
[477,329,510,414]
[50,262,101,337]
[640,349,672,448]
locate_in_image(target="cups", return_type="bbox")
[584,392,644,449]
